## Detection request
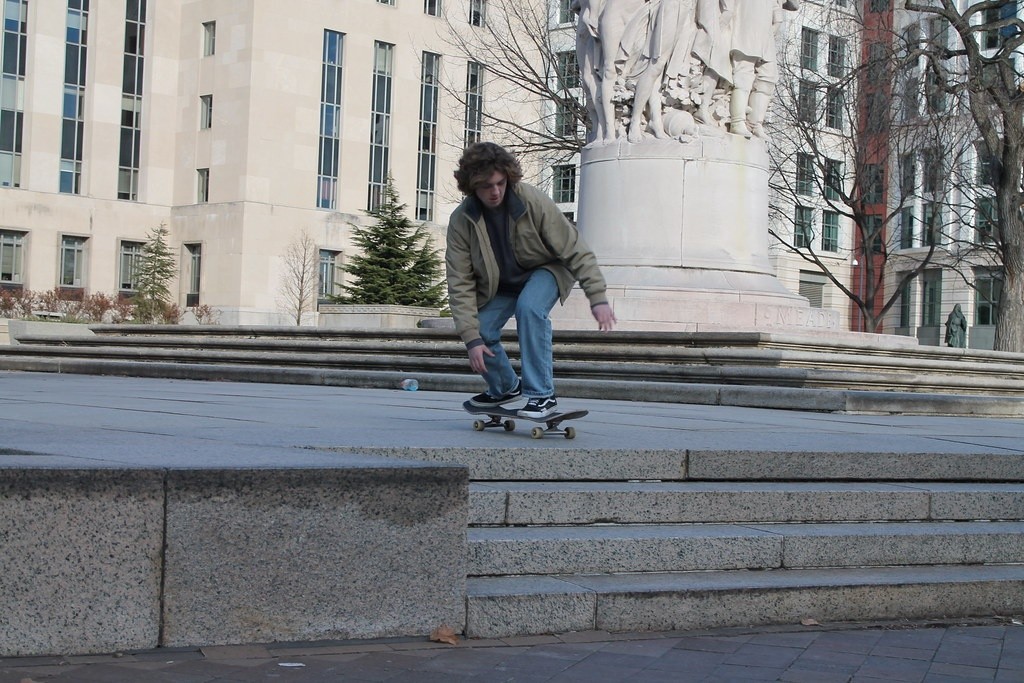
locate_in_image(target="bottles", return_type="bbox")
[400,379,419,391]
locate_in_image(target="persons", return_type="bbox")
[572,0,800,144]
[944,304,968,348]
[445,142,617,418]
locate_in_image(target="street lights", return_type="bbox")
[853,257,863,332]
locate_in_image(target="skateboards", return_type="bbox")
[463,401,589,439]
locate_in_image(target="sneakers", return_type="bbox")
[518,393,559,419]
[469,386,522,408]
